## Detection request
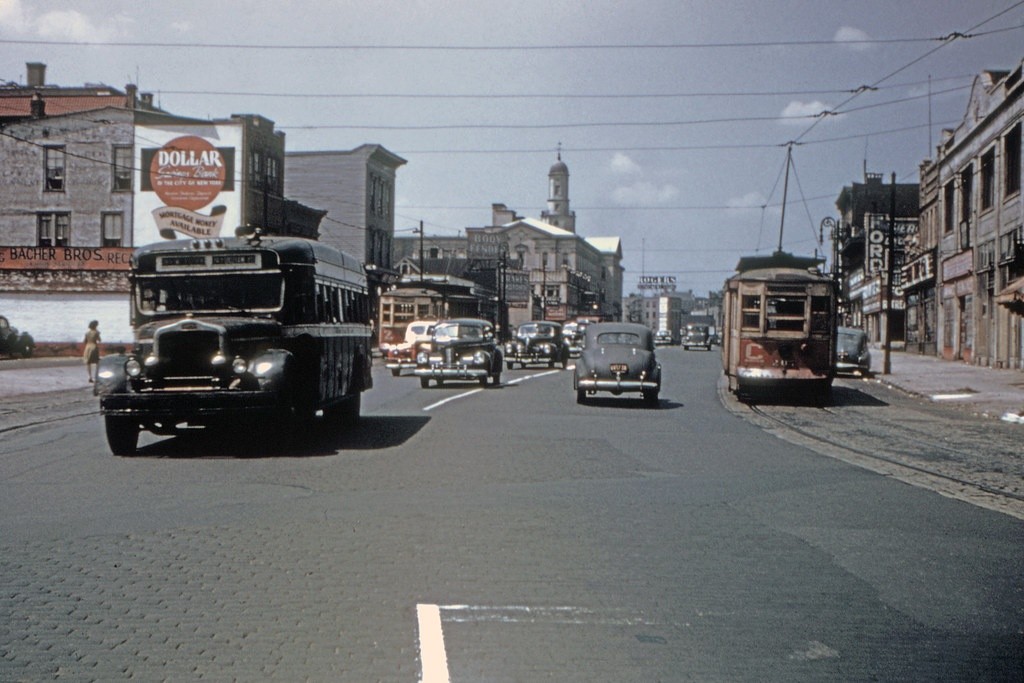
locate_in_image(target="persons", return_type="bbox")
[83,320,102,382]
[508,324,514,341]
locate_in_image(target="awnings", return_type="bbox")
[993,277,1024,304]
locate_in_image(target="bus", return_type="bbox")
[379,288,444,365]
[719,270,838,401]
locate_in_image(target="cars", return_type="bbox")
[412,318,501,387]
[574,323,661,403]
[836,326,872,376]
[502,320,568,368]
[681,325,712,349]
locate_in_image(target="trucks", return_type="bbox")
[94,229,378,458]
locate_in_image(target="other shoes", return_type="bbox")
[89,378,94,383]
